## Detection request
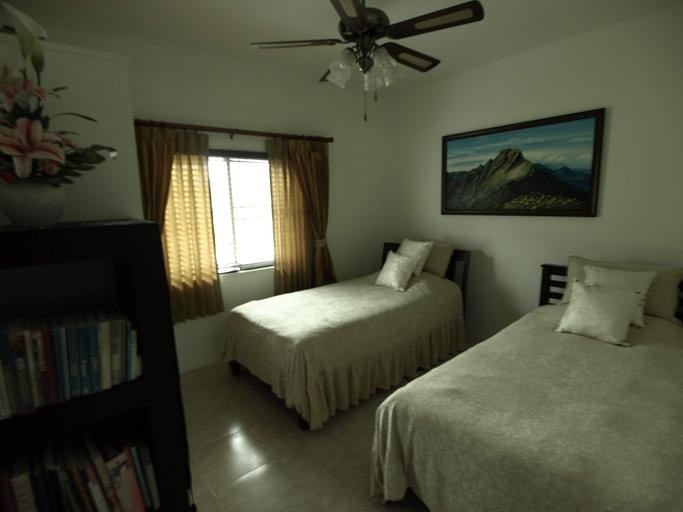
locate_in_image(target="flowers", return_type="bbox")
[1,0,119,186]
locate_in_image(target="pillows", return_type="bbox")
[559,253,683,348]
[375,238,455,291]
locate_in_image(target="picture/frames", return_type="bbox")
[440,106,604,219]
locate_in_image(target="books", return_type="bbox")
[0,310,143,422]
[1,435,161,511]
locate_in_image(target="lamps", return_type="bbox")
[328,37,399,91]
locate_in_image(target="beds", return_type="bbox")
[225,243,469,429]
[374,264,683,511]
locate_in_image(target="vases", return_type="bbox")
[1,176,66,224]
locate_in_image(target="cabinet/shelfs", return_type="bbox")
[3,216,197,511]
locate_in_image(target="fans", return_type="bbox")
[249,0,486,82]
